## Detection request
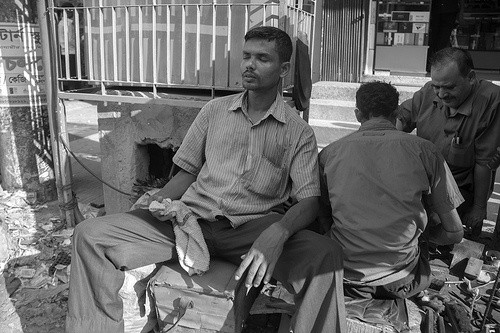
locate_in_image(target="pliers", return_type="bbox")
[447,291,499,329]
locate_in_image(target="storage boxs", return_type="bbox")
[144,267,266,333]
[279,289,423,333]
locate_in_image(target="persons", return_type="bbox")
[318,81,465,301]
[395,46,500,235]
[58,3,77,89]
[66,26,346,333]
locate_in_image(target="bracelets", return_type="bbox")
[473,203,487,210]
